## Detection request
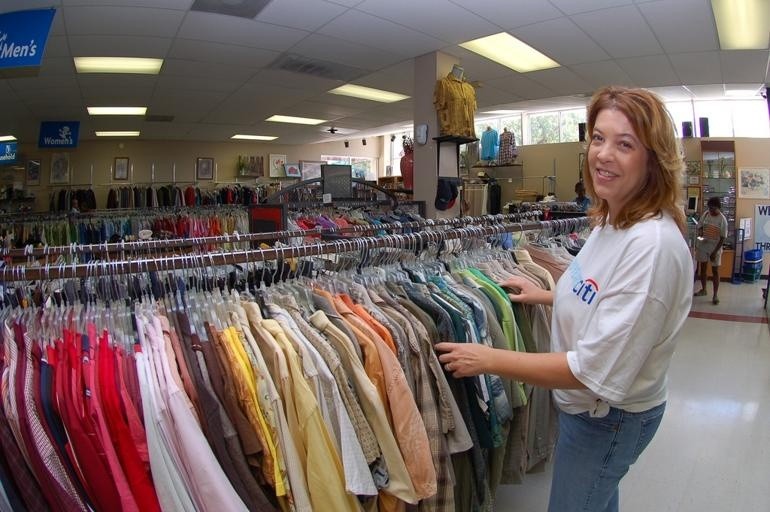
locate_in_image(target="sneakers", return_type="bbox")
[695,290,707,296]
[713,298,719,305]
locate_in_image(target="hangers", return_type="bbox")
[1,205,252,226]
[1,211,606,355]
[51,184,93,199]
[287,199,422,231]
[108,181,289,195]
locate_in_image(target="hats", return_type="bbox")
[435,179,451,210]
[448,181,458,209]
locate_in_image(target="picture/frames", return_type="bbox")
[114,158,129,178]
[301,161,328,185]
[26,160,40,185]
[197,157,214,180]
[49,156,68,183]
[268,153,286,176]
[285,164,301,178]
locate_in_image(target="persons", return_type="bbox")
[574,182,589,209]
[435,87,692,512]
[499,127,516,164]
[693,195,728,304]
[439,65,477,136]
[481,127,498,161]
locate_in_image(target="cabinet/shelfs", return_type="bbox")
[700,140,738,280]
